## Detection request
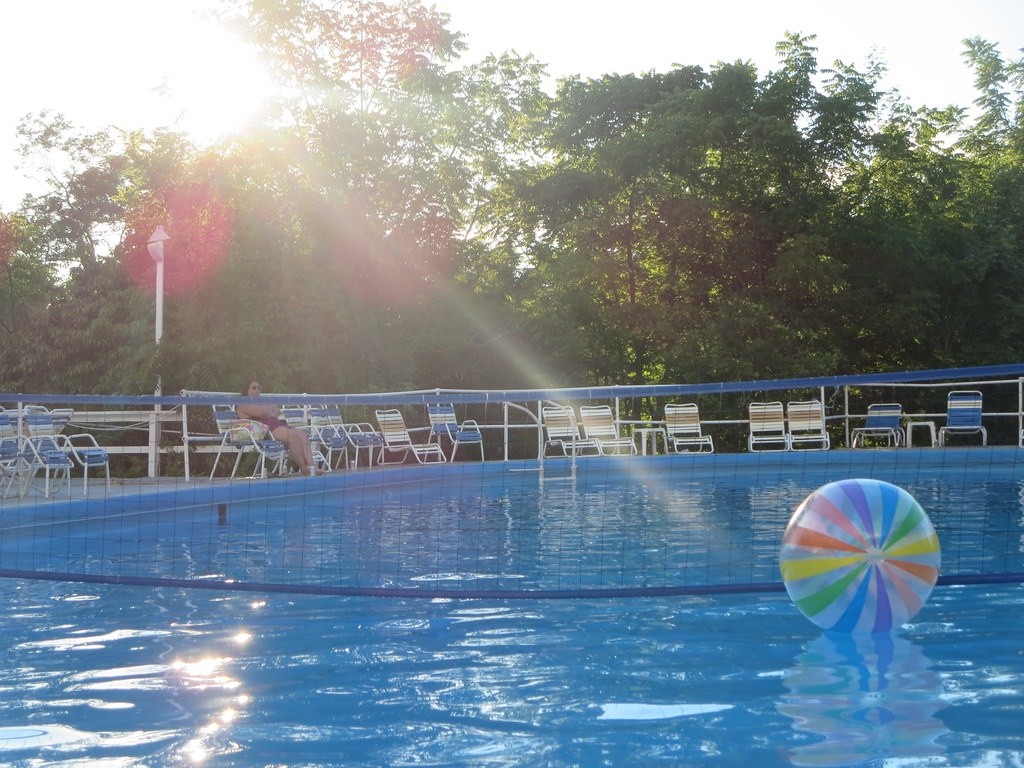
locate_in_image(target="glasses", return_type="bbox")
[251,385,261,390]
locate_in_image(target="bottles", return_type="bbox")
[349,457,356,472]
[308,463,317,477]
[263,466,268,479]
[282,463,288,477]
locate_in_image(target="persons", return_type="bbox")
[234,380,324,477]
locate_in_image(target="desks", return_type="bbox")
[907,421,937,448]
[631,428,669,456]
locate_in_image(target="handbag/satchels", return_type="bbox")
[228,419,270,444]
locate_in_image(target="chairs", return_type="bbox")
[852,403,903,449]
[664,403,714,454]
[324,404,385,466]
[280,405,349,471]
[938,390,988,447]
[232,403,332,473]
[542,405,602,457]
[787,400,830,452]
[0,405,111,499]
[423,401,485,462]
[374,408,447,465]
[308,404,373,470]
[580,404,638,457]
[208,405,287,479]
[747,401,790,453]
